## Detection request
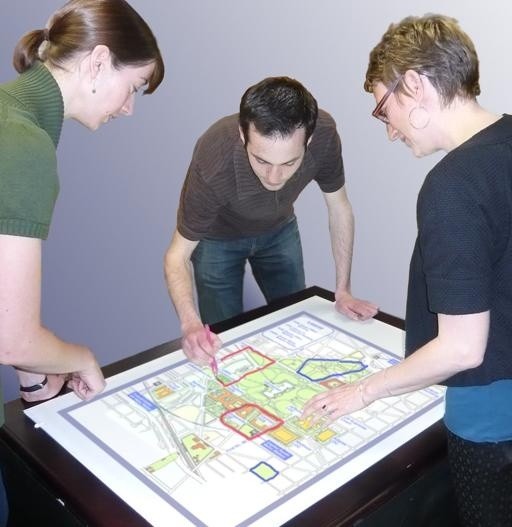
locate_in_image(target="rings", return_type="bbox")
[321,405,329,413]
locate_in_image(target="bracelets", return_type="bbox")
[19,374,48,392]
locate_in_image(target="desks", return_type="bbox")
[1,284,448,527]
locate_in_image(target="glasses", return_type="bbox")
[371,74,403,125]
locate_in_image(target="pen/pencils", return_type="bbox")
[205,324,220,379]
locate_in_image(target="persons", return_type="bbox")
[164,76,380,367]
[0,0,165,527]
[296,12,512,527]
